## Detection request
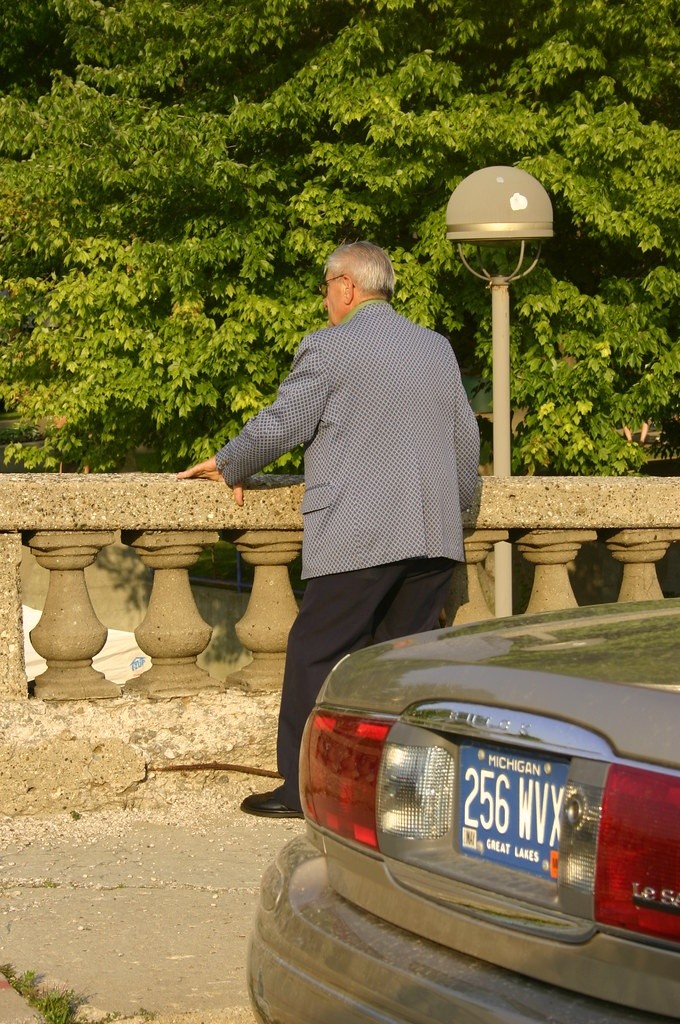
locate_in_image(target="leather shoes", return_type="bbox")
[241,791,304,819]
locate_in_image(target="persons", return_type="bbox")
[176,241,481,818]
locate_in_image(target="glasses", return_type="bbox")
[319,274,355,297]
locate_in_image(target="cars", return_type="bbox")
[239,600,679,1024]
